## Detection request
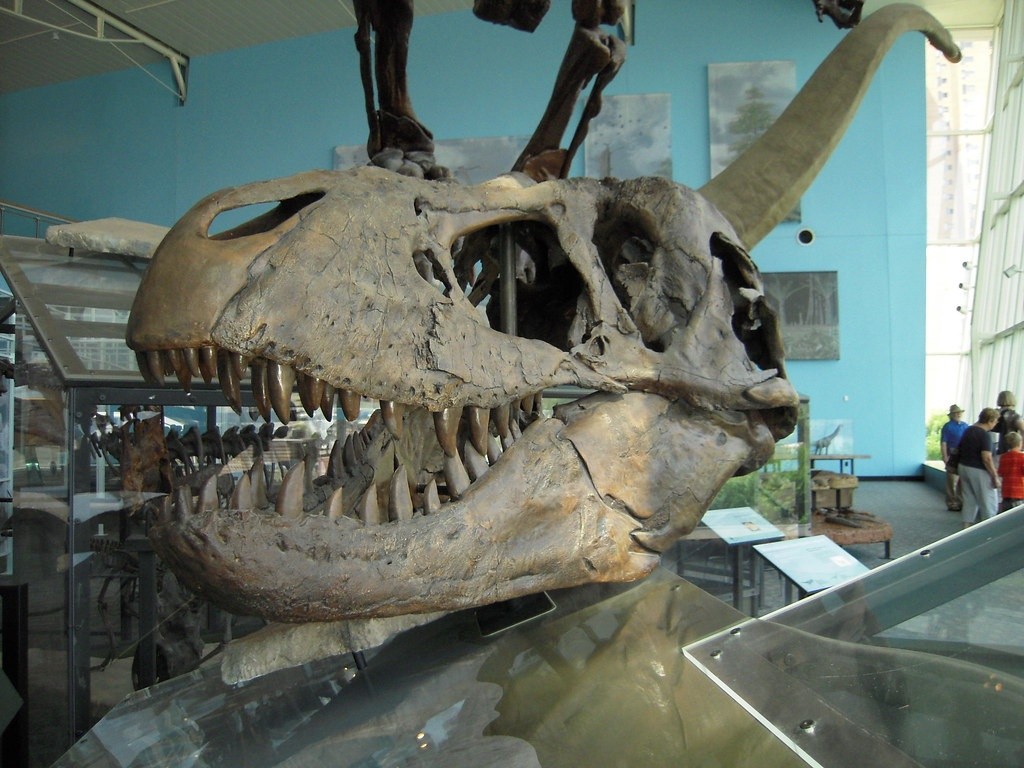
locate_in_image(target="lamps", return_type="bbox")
[1005,265,1024,278]
[957,306,972,316]
[962,261,977,271]
[959,282,975,291]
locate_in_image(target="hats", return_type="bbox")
[947,404,965,416]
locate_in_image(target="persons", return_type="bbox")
[940,405,970,511]
[991,391,1024,516]
[957,408,1002,530]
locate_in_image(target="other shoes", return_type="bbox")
[949,505,962,511]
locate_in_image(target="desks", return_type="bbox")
[811,453,871,473]
